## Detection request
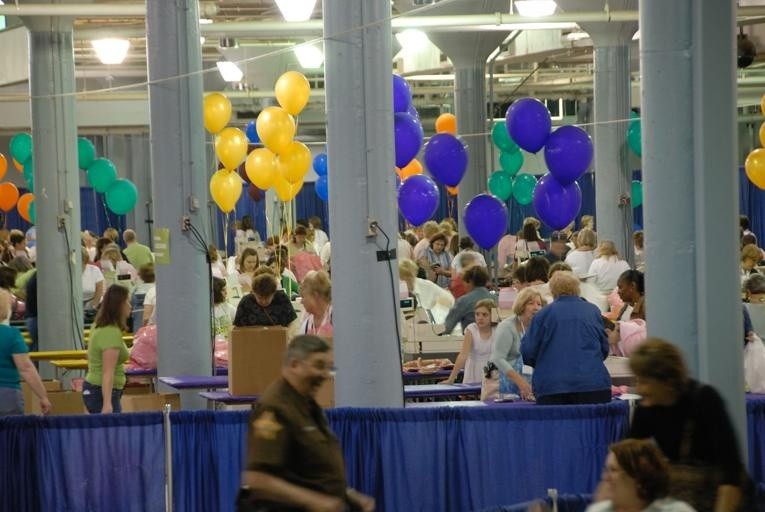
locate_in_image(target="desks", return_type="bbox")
[396,352,531,411]
[8,294,258,414]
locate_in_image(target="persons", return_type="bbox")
[208,215,336,340]
[1,213,156,415]
[396,213,648,404]
[738,215,764,394]
[592,338,763,512]
[235,333,376,512]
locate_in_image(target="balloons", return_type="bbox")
[506,94,552,154]
[391,74,468,229]
[628,107,644,159]
[485,121,537,208]
[462,195,513,257]
[744,86,765,195]
[530,174,586,232]
[545,124,597,184]
[0,64,139,229]
[630,175,650,211]
[205,68,327,217]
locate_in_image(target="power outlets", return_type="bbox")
[366,216,377,236]
[57,215,65,228]
[182,215,191,231]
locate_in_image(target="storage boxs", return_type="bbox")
[226,323,287,396]
